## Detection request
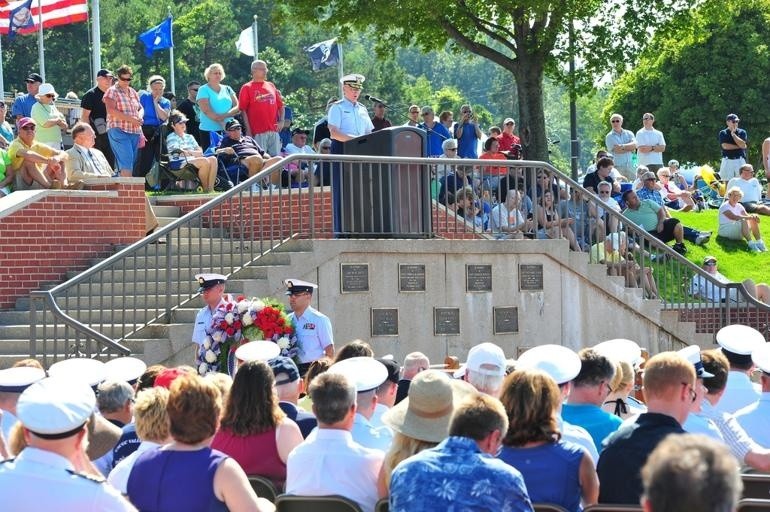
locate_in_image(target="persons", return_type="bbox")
[719,113,747,185]
[192,273,335,375]
[527,150,770,306]
[370,101,391,133]
[403,105,534,239]
[1,324,770,512]
[327,74,375,239]
[606,113,666,179]
[1,60,331,196]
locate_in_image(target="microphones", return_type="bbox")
[364,93,387,108]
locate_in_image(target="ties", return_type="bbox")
[88,149,104,174]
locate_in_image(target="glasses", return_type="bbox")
[118,74,131,81]
[682,382,696,402]
[22,126,34,130]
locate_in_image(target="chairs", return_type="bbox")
[157,125,200,194]
[203,131,249,189]
[248,475,277,504]
[739,472,770,498]
[734,497,770,510]
[376,495,390,511]
[277,491,362,511]
[527,502,566,512]
[580,503,644,511]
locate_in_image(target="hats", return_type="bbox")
[97,69,113,77]
[678,324,770,378]
[727,114,739,122]
[16,117,36,128]
[503,118,515,126]
[452,338,642,386]
[703,256,716,263]
[340,74,365,89]
[642,172,655,181]
[292,128,308,136]
[0,357,191,439]
[169,115,188,124]
[283,279,318,294]
[381,369,478,443]
[234,340,400,393]
[34,83,58,99]
[195,274,227,291]
[24,73,42,83]
[226,118,242,130]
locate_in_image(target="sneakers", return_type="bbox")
[651,243,687,263]
[756,241,768,252]
[748,243,762,253]
[695,232,712,246]
[68,182,83,189]
[51,180,61,189]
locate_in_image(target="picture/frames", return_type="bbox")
[369,307,399,337]
[518,263,545,292]
[433,307,461,336]
[517,346,534,358]
[465,263,493,292]
[339,262,370,294]
[397,263,426,293]
[493,306,521,335]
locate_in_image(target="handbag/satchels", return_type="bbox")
[94,118,106,135]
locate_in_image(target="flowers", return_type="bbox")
[198,292,301,375]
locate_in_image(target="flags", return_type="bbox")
[235,25,255,57]
[139,18,172,58]
[305,38,339,73]
[8,1,35,38]
[0,0,88,34]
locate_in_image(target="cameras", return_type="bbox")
[469,113,474,119]
